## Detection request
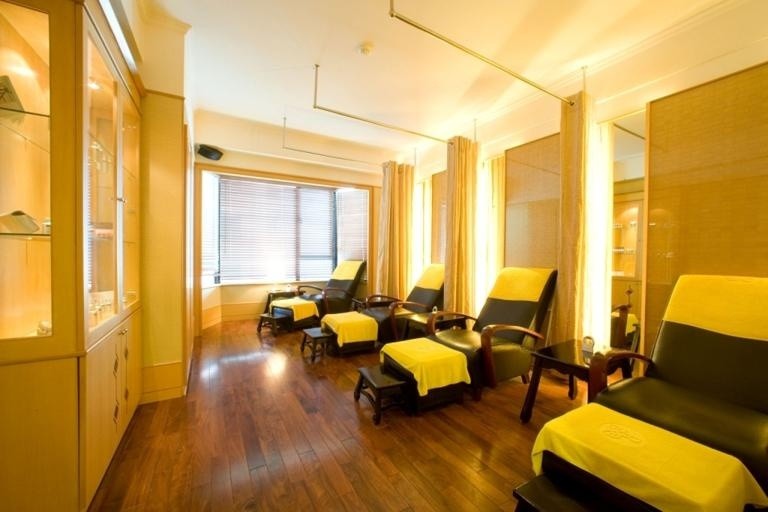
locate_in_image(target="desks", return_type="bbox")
[401,310,466,342]
[352,297,394,311]
[518,336,633,423]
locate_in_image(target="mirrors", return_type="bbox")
[611,109,641,374]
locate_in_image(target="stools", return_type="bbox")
[321,311,379,356]
[379,337,470,417]
[512,475,599,511]
[300,327,332,362]
[258,312,289,337]
[352,363,412,426]
[530,402,767,511]
[270,298,316,333]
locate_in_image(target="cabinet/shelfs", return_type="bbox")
[0,1,141,511]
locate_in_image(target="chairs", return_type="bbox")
[362,261,445,353]
[298,259,367,321]
[585,273,767,495]
[426,265,559,402]
